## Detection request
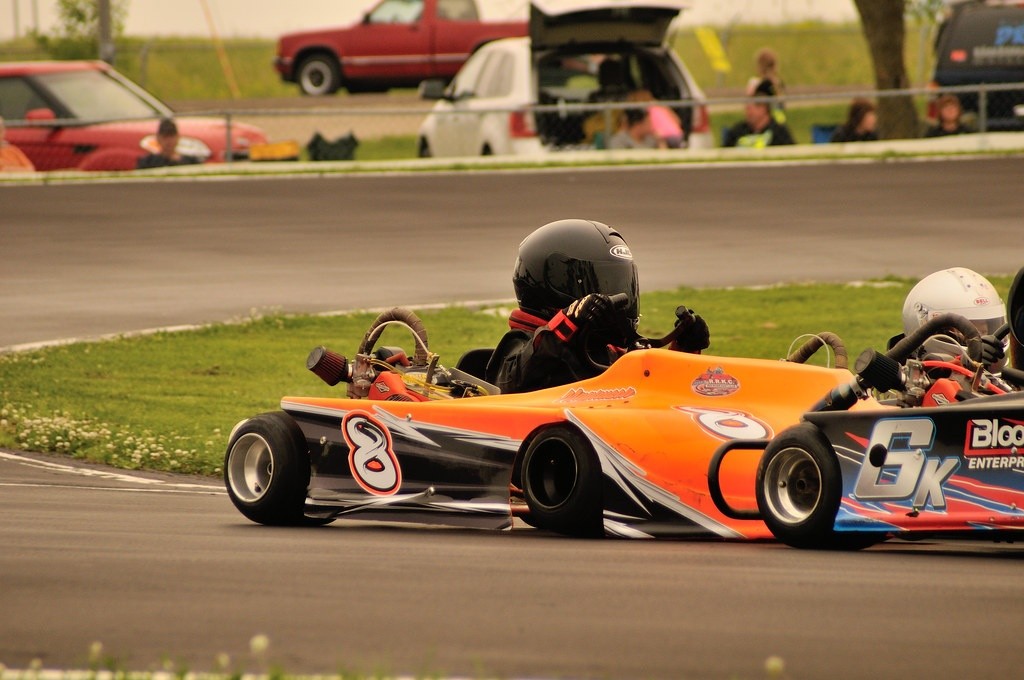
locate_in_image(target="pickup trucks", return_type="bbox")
[275,1,531,97]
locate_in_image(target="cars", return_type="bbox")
[0,57,270,174]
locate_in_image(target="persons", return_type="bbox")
[137,120,197,169]
[902,267,1010,394]
[486,220,710,395]
[581,52,975,149]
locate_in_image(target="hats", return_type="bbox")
[845,98,875,129]
[937,93,961,121]
[157,119,178,136]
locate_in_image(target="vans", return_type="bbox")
[417,3,719,152]
[932,0,1024,126]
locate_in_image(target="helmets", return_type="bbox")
[902,266,1006,337]
[512,218,640,320]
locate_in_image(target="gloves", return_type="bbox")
[567,293,613,327]
[674,315,710,352]
[978,334,1005,366]
